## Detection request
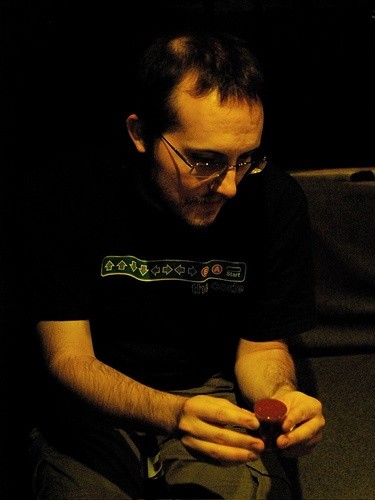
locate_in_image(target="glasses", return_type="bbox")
[158,132,270,180]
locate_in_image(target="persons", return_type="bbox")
[26,34,326,500]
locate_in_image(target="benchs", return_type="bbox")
[289,167,375,500]
